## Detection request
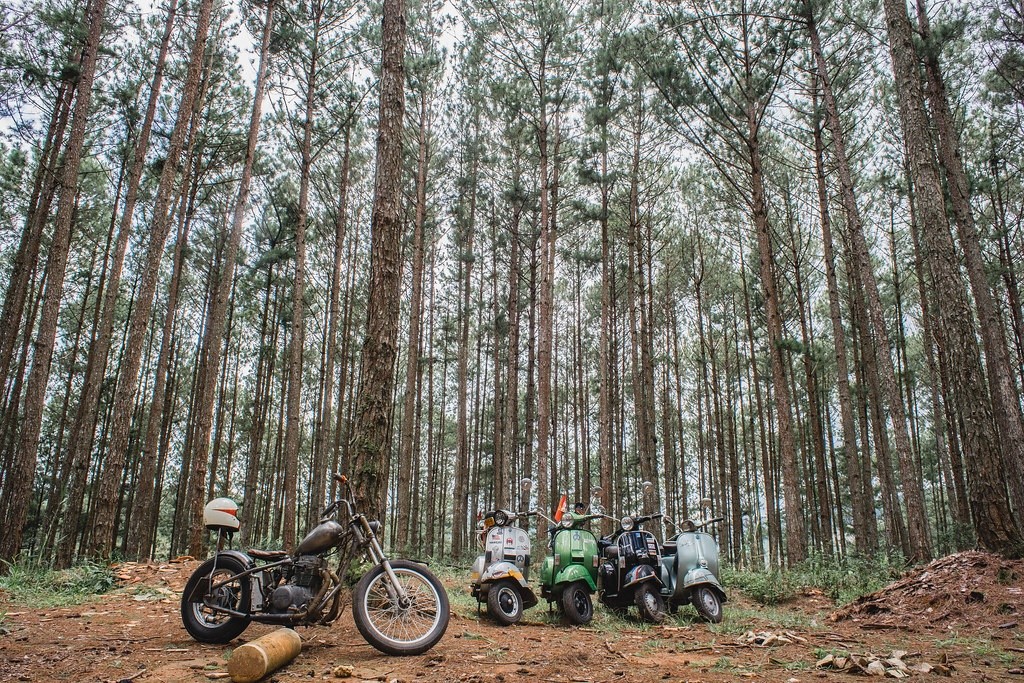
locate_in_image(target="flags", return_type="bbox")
[555,489,567,522]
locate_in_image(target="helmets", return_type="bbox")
[203,498,241,532]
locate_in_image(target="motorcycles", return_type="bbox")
[532,486,606,626]
[597,482,668,624]
[180,473,451,657]
[468,477,539,624]
[660,497,728,623]
